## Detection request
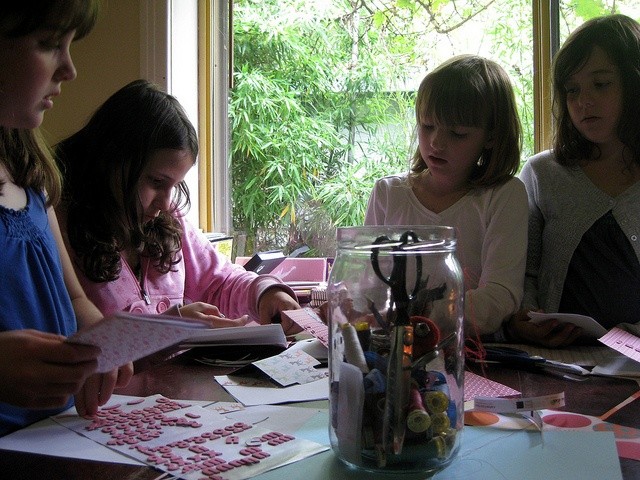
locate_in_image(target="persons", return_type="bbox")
[490,13,640,348]
[50,78,307,337]
[0,0,136,436]
[363,53,530,336]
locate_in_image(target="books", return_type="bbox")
[178,323,295,352]
[236,253,326,302]
[202,231,233,261]
[592,321,640,378]
[62,310,207,376]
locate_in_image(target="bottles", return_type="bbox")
[326,225,464,475]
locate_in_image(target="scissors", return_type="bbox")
[482,345,590,384]
[370,231,421,457]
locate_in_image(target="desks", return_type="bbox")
[0,343,638,480]
